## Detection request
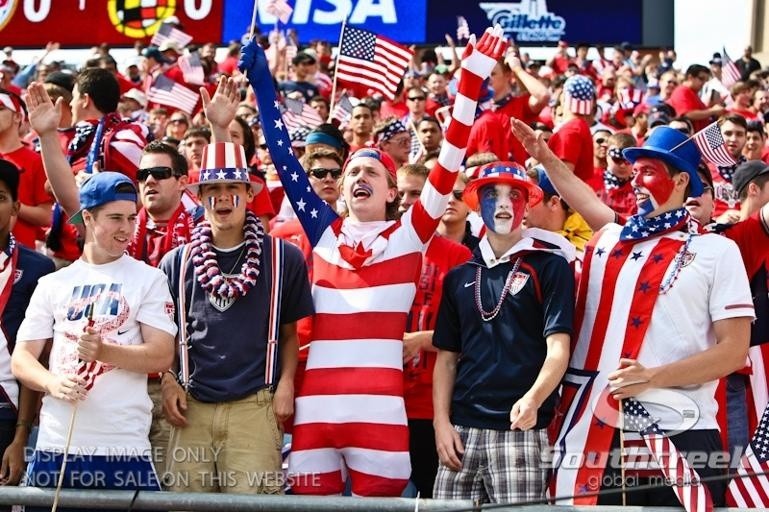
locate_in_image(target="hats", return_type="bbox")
[122,87,147,107]
[648,105,671,117]
[63,170,138,226]
[527,164,557,197]
[561,73,597,116]
[342,148,400,181]
[619,40,636,54]
[526,59,542,70]
[0,93,26,122]
[695,158,714,184]
[289,52,313,65]
[619,125,705,201]
[184,141,265,200]
[457,160,545,213]
[646,110,670,127]
[729,157,769,198]
[0,159,24,205]
[40,69,76,94]
[138,45,173,64]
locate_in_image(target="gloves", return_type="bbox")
[236,34,272,89]
[457,21,509,86]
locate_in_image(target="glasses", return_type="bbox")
[133,164,182,184]
[165,117,189,125]
[701,180,713,190]
[594,137,607,145]
[307,166,345,181]
[405,95,427,103]
[386,135,413,148]
[611,154,632,165]
[256,142,270,151]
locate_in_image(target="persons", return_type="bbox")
[0,22,769,511]
